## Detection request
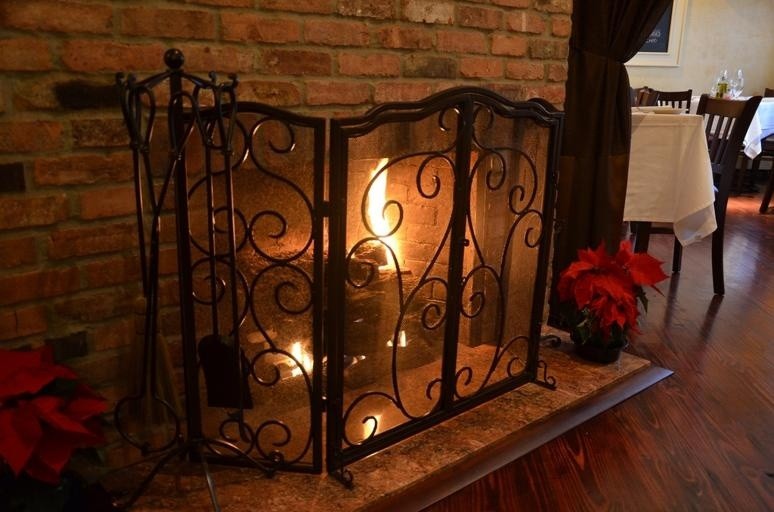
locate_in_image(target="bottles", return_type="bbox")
[710,68,745,99]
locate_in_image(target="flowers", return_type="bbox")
[1,349,116,512]
[551,230,672,348]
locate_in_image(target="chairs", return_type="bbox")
[628,85,774,295]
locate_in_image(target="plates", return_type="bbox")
[653,107,689,115]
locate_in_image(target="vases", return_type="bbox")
[573,335,629,366]
